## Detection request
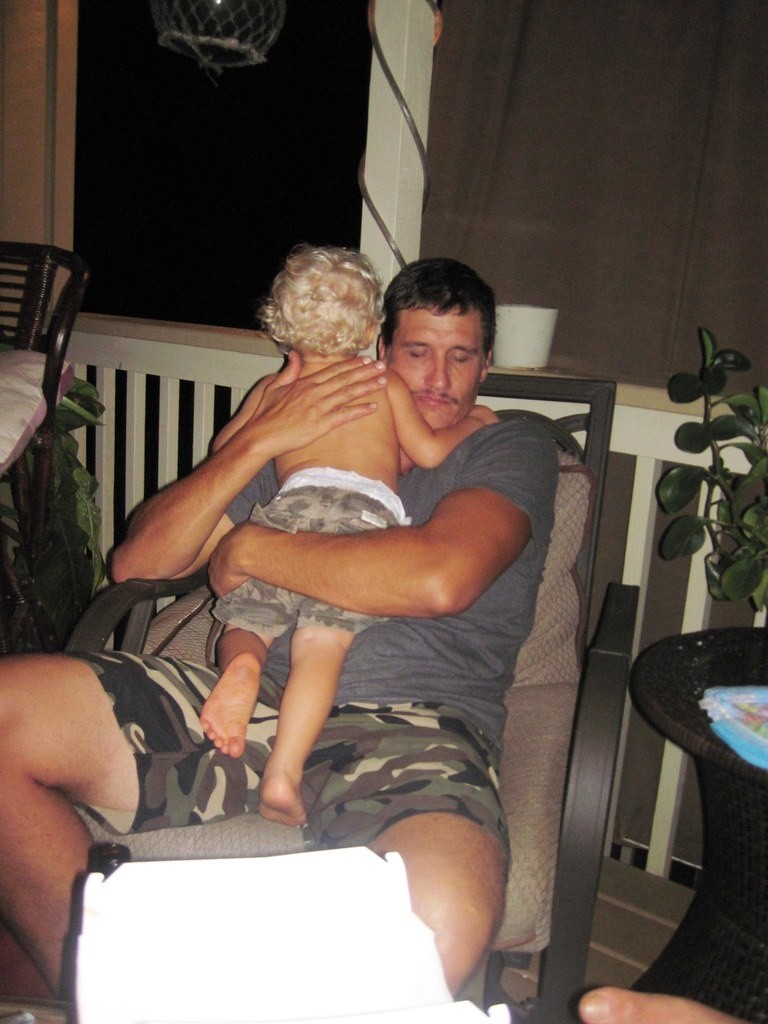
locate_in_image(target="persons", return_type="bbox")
[202,247,501,827]
[0,257,562,1000]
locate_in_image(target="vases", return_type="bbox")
[491,304,560,368]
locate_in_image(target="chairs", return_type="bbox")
[61,373,640,1023]
[0,241,93,654]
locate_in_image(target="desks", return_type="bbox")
[629,625,768,1024]
